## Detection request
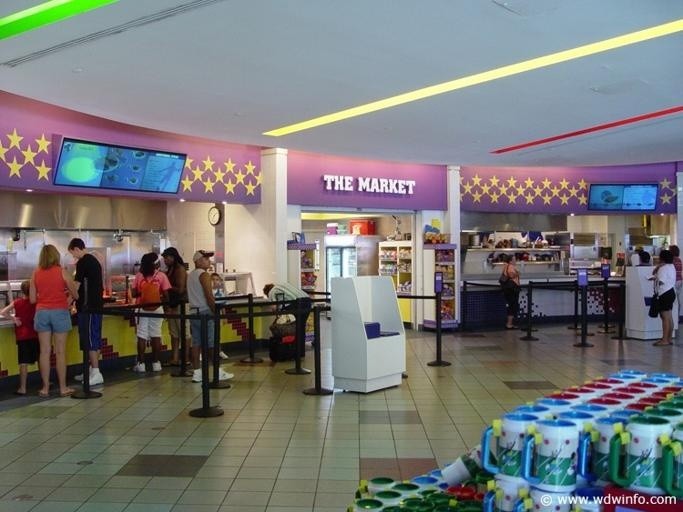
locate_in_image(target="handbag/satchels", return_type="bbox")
[167,268,189,306]
[648,292,660,319]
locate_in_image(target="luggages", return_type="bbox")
[268,292,297,364]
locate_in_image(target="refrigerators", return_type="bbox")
[324,234,383,320]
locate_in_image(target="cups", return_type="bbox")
[479,368,683,511]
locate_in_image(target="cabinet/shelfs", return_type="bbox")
[423,244,457,328]
[460,248,570,282]
[287,243,316,342]
[378,239,412,292]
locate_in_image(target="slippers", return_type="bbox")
[14,380,74,396]
[505,325,519,330]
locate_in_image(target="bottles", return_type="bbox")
[351,453,483,512]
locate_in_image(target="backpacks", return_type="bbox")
[499,264,522,294]
[137,271,160,305]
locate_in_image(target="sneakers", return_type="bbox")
[131,361,146,373]
[72,369,103,385]
[217,368,234,380]
[218,350,228,360]
[191,369,202,383]
[150,360,162,372]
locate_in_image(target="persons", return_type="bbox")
[653,250,676,346]
[263,282,311,360]
[627,245,682,321]
[129,247,234,382]
[0,280,53,395]
[500,255,521,329]
[30,245,80,398]
[68,239,105,385]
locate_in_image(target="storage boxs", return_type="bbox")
[349,219,377,235]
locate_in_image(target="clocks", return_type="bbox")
[208,206,221,226]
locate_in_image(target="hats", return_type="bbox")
[160,247,177,257]
[192,249,214,263]
